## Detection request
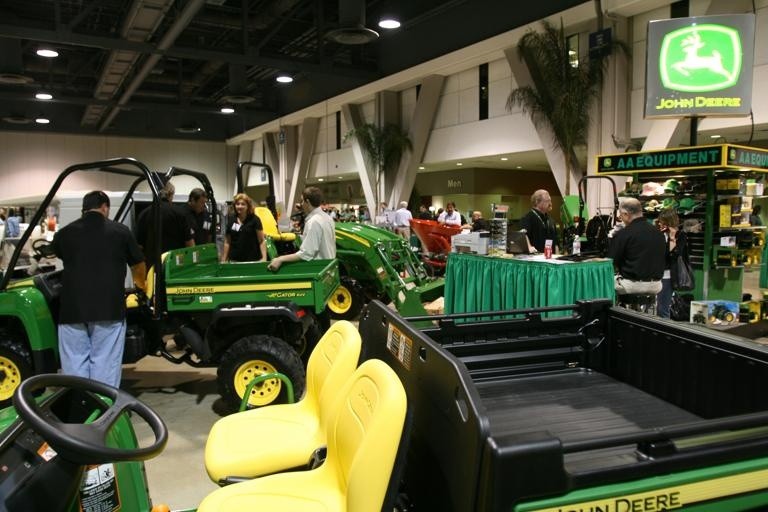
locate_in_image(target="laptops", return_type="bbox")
[556,250,599,262]
[507,231,540,254]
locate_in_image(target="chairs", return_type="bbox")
[195,356,416,512]
[205,317,364,488]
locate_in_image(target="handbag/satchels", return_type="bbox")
[671,254,697,292]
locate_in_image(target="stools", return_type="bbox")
[615,293,657,313]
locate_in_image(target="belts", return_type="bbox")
[625,276,663,283]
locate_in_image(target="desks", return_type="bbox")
[443,249,618,324]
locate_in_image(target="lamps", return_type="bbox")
[35,41,60,58]
[377,5,402,30]
[220,104,234,115]
[275,70,293,84]
[35,114,49,124]
[33,88,53,101]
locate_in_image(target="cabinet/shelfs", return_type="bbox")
[713,193,766,270]
[0,203,56,271]
[626,191,704,239]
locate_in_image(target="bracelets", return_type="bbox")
[135,280,147,288]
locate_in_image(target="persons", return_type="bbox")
[380,202,392,233]
[392,201,413,243]
[181,188,213,245]
[519,189,561,254]
[136,180,194,274]
[266,187,338,338]
[653,206,690,321]
[0,207,21,268]
[607,197,666,296]
[222,193,267,264]
[749,205,764,246]
[290,202,369,237]
[46,189,150,390]
[406,201,492,239]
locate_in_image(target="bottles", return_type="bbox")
[573,235,580,256]
